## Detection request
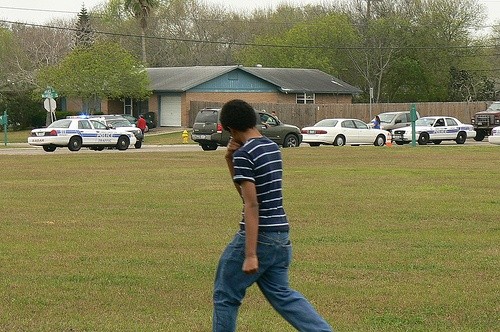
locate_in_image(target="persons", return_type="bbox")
[261,108,278,122]
[213,99,332,332]
[371,116,380,129]
[137,115,146,142]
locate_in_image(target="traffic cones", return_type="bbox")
[386,133,391,147]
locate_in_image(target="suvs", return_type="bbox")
[367,110,420,132]
[87,115,144,151]
[470,102,500,141]
[189,106,304,151]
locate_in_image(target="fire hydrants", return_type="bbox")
[182,130,189,144]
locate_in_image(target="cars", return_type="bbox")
[391,116,476,145]
[122,114,149,133]
[27,115,137,152]
[301,118,392,147]
